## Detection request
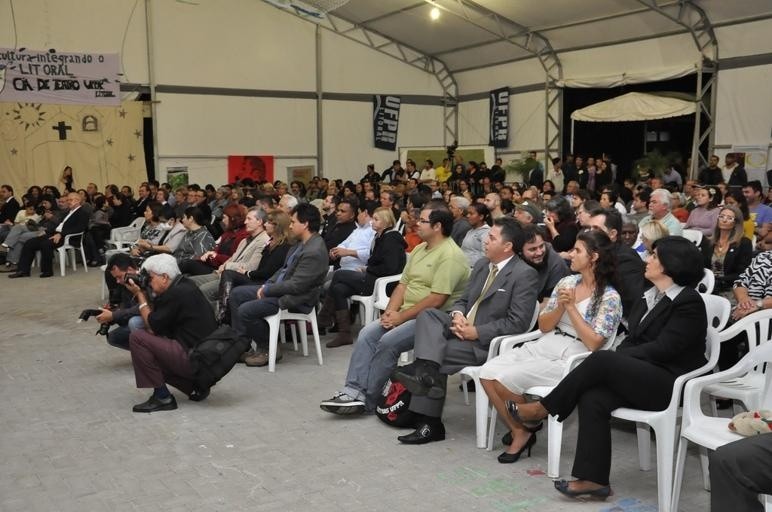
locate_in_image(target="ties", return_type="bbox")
[464,266,497,327]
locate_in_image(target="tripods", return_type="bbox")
[445,154,456,171]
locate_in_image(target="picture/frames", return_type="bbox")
[398,144,497,172]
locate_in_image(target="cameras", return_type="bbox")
[79,309,115,339]
[124,267,151,290]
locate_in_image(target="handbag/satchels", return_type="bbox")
[375,378,413,428]
[719,288,748,323]
[186,324,251,390]
[179,258,212,275]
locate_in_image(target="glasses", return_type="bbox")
[717,213,736,222]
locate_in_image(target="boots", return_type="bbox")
[217,280,233,322]
[318,295,353,347]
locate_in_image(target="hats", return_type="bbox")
[691,179,704,188]
[511,199,543,219]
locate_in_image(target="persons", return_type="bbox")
[1,152,771,511]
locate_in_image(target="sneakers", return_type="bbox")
[238,344,283,366]
[83,259,105,267]
[0,243,11,253]
[460,378,475,392]
[0,262,19,272]
[319,392,365,414]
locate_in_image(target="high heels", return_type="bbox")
[498,433,536,463]
[502,422,543,445]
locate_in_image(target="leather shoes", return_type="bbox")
[398,423,446,443]
[189,383,210,401]
[9,269,31,278]
[40,271,53,277]
[554,479,610,501]
[506,400,542,425]
[396,371,445,399]
[133,395,177,412]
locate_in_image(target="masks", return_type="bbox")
[160,220,173,231]
